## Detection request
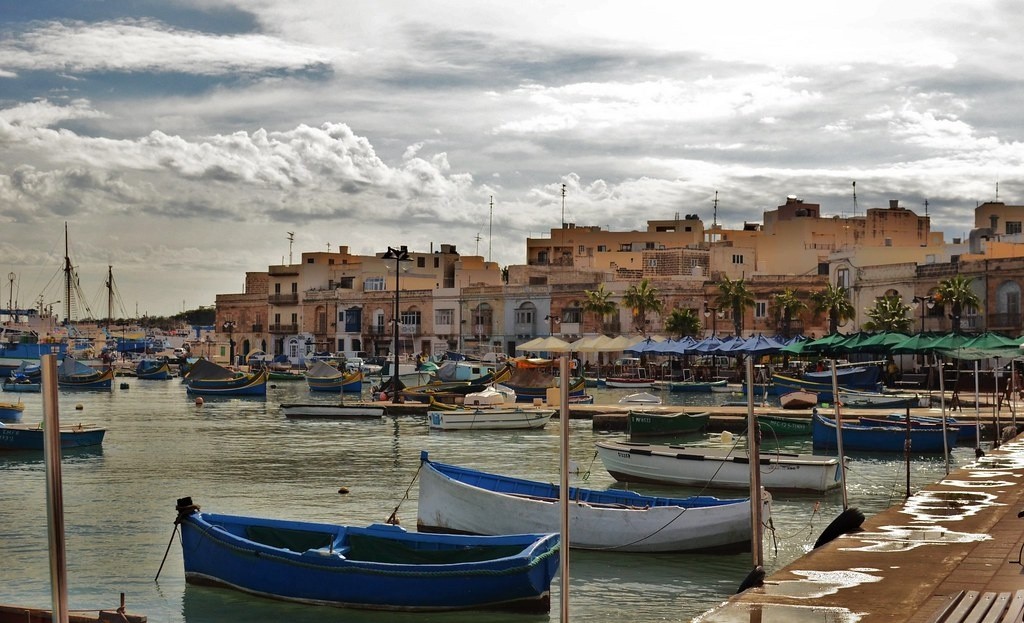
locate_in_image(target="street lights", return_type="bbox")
[544,314,561,336]
[910,295,936,374]
[704,305,724,369]
[381,246,414,403]
[223,320,237,364]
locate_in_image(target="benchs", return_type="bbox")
[318,543,351,554]
[922,588,1024,623]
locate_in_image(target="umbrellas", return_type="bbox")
[514,329,1024,380]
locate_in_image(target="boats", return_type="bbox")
[858,412,987,441]
[0,600,150,623]
[415,447,772,552]
[1,422,107,450]
[627,409,712,436]
[426,403,556,431]
[810,406,959,453]
[593,440,853,496]
[173,494,561,611]
[2,348,930,416]
[0,396,25,420]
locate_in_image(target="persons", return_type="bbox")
[888,360,899,389]
